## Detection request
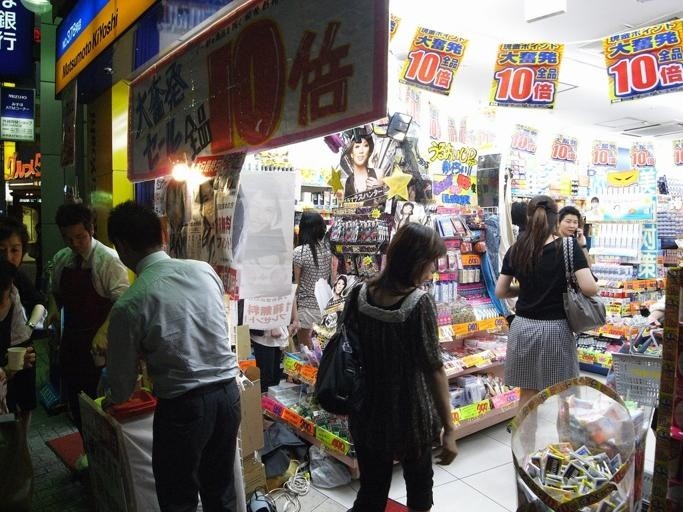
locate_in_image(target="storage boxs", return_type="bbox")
[239,365,268,500]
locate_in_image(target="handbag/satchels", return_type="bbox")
[561,235,606,334]
[0,412,35,511]
[313,284,372,416]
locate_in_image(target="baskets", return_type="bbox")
[612,323,663,408]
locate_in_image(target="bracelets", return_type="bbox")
[579,244,587,251]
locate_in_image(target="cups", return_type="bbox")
[8,347,26,371]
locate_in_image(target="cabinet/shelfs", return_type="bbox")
[571,278,667,376]
[261,316,519,481]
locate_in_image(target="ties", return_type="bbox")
[75,254,84,268]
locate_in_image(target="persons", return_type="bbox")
[329,276,348,303]
[342,134,386,197]
[291,211,335,354]
[512,201,528,239]
[587,196,600,222]
[0,257,38,510]
[0,212,52,505]
[552,207,592,270]
[41,201,133,476]
[197,179,218,266]
[335,221,459,512]
[494,195,602,463]
[396,201,421,233]
[248,299,300,395]
[103,198,247,511]
[167,181,186,259]
[240,188,283,234]
